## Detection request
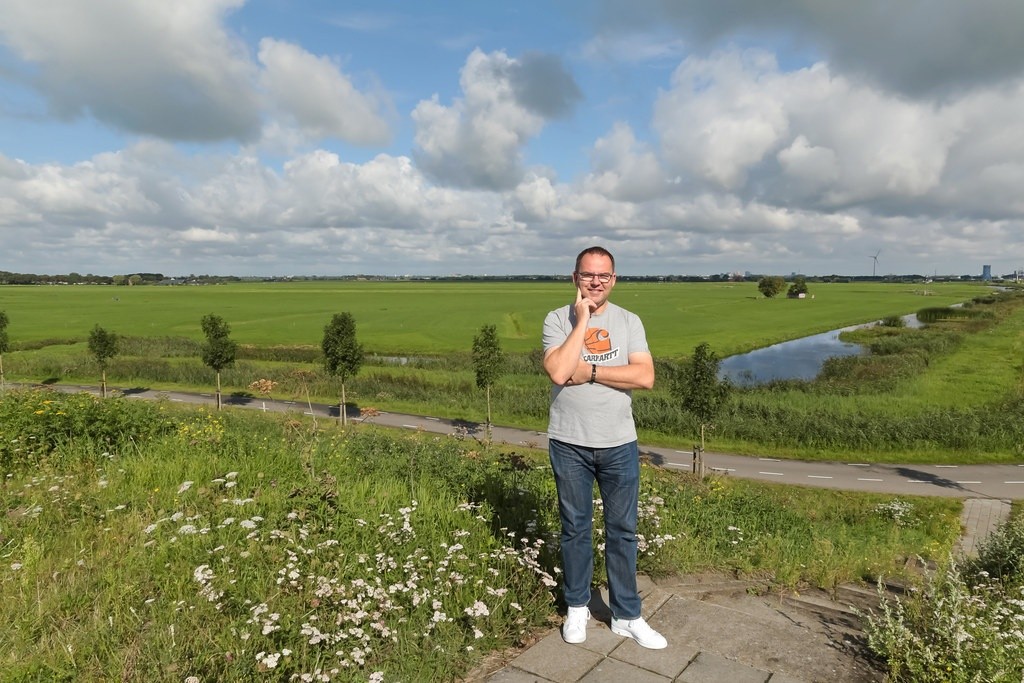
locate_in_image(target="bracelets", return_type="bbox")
[591,363,596,383]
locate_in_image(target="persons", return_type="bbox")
[542,247,671,650]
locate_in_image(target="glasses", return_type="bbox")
[575,272,613,283]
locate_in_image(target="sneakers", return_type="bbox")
[562,605,591,643]
[610,615,667,649]
